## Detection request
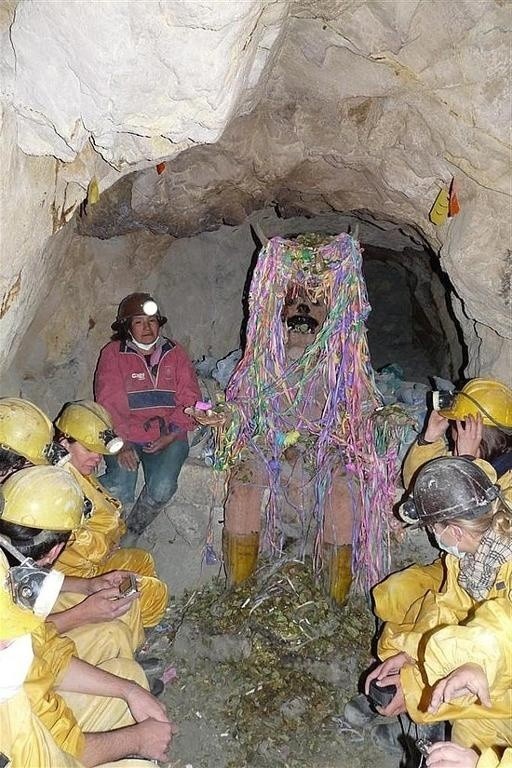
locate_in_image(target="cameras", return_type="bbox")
[416,738,433,758]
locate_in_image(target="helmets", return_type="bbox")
[0,560,44,643]
[55,396,85,539]
[110,292,168,332]
[53,399,125,456]
[436,376,512,431]
[0,464,85,532]
[398,455,502,530]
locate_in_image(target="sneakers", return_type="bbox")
[139,651,164,699]
[370,718,446,758]
[344,690,380,728]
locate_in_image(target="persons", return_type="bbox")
[95,293,203,548]
[1,396,180,768]
[185,230,418,617]
[345,375,512,768]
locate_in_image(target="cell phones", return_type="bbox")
[113,575,138,598]
